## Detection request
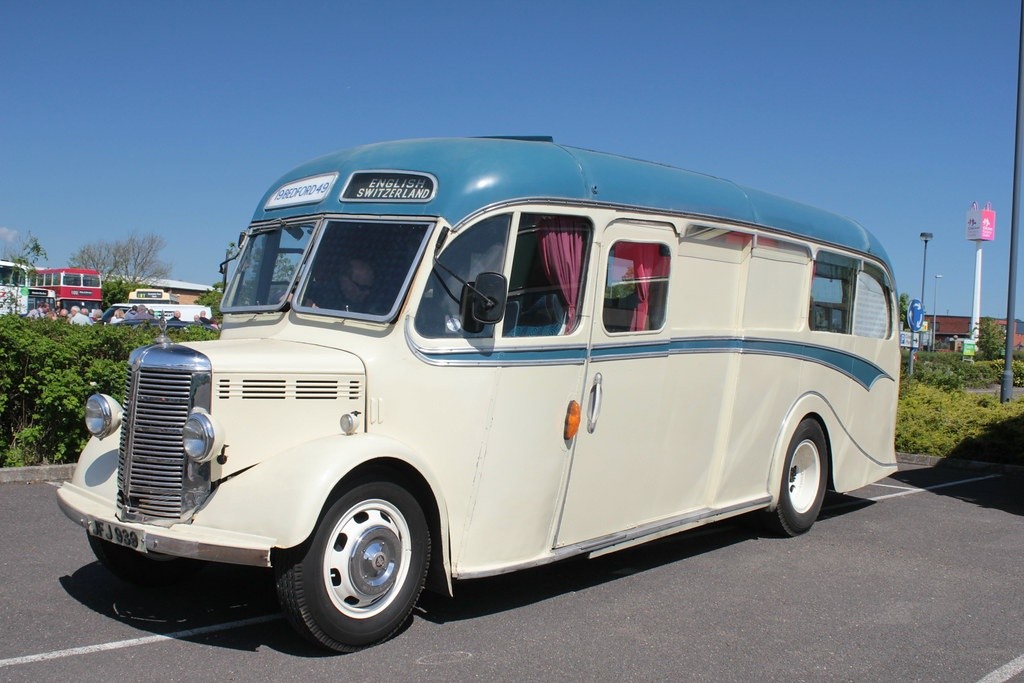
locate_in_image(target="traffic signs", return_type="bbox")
[899,331,919,348]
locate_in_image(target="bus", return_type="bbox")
[128,288,179,304]
[0,260,29,318]
[25,269,102,322]
[57,134,901,653]
[99,303,213,332]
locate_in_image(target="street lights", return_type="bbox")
[918,232,934,350]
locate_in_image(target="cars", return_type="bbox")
[116,318,214,332]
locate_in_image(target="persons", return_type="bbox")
[26,299,224,335]
